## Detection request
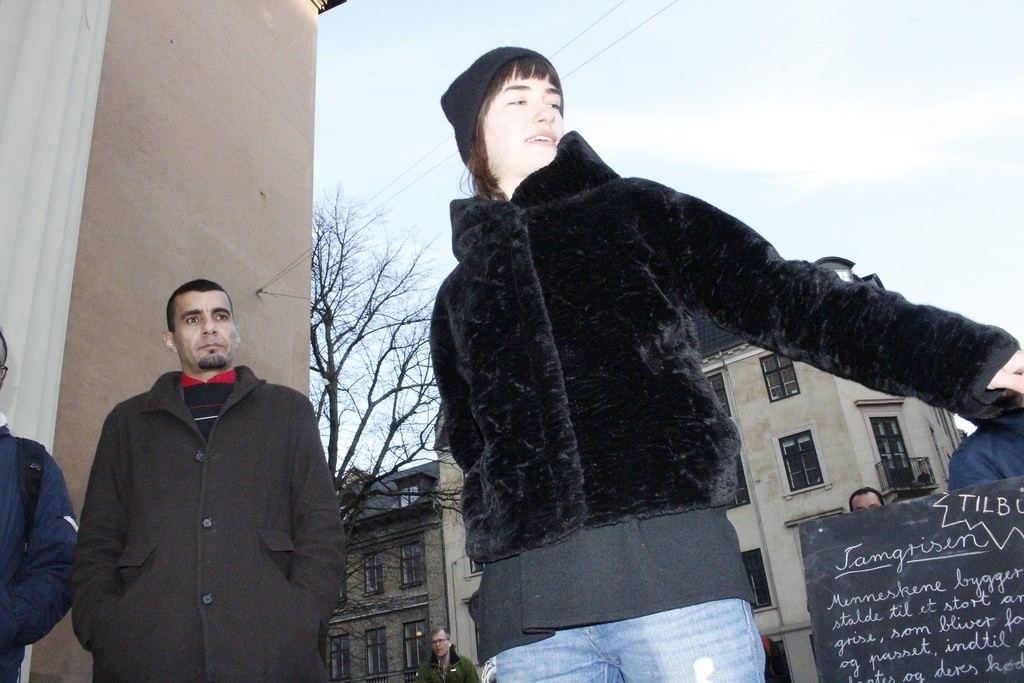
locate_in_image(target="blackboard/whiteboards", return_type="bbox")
[798,474,1024,683]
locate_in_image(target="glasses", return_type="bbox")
[0,364,8,379]
[431,639,449,645]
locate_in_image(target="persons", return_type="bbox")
[0,332,78,683]
[72,278,348,683]
[413,627,480,683]
[429,46,1024,683]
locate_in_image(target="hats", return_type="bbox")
[441,47,563,173]
